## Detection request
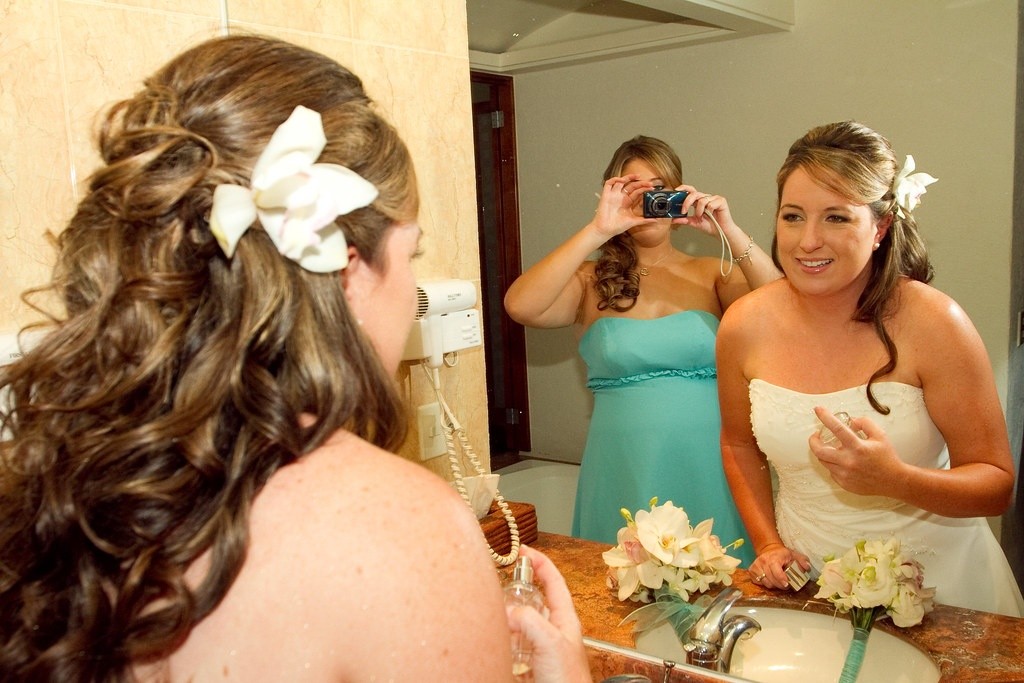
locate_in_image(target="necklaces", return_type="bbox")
[633,246,673,276]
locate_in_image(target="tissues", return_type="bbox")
[436,473,540,568]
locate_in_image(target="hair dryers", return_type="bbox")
[411,279,478,369]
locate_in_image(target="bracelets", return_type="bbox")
[729,235,754,266]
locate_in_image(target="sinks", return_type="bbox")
[631,604,942,683]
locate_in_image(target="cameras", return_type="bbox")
[643,191,690,218]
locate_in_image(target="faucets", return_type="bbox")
[684,585,762,674]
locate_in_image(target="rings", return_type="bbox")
[623,188,630,195]
[705,196,711,202]
[755,573,766,582]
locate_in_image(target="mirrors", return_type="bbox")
[206,1,1022,626]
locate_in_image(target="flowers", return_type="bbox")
[814,528,938,683]
[205,99,374,274]
[890,154,940,220]
[598,495,744,651]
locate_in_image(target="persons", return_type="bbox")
[0,35,593,683]
[716,119,1024,621]
[503,134,786,571]
